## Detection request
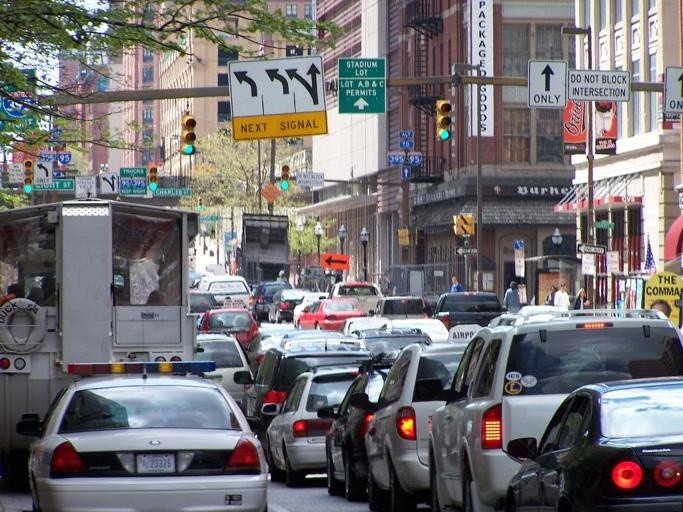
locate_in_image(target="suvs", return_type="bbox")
[428,308,681,507]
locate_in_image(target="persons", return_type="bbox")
[545,287,558,306]
[651,299,671,325]
[450,275,462,293]
[503,282,521,313]
[574,289,590,309]
[554,283,572,310]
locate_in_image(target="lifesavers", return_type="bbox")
[0,299,47,353]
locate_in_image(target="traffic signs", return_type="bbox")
[119,168,146,194]
[455,248,478,256]
[530,64,565,107]
[573,242,606,256]
[593,219,614,231]
[33,178,74,191]
[667,70,682,117]
[153,187,192,196]
[296,172,324,188]
[337,58,386,112]
[199,214,217,221]
[228,63,328,141]
[569,70,629,100]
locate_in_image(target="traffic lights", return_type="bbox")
[179,115,196,157]
[149,167,157,190]
[22,159,36,196]
[437,100,449,141]
[280,166,289,187]
[196,198,203,212]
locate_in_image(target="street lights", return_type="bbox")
[559,23,601,319]
[336,221,348,260]
[313,217,323,270]
[357,228,370,283]
[295,214,303,277]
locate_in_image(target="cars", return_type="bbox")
[20,360,275,507]
[502,371,682,507]
